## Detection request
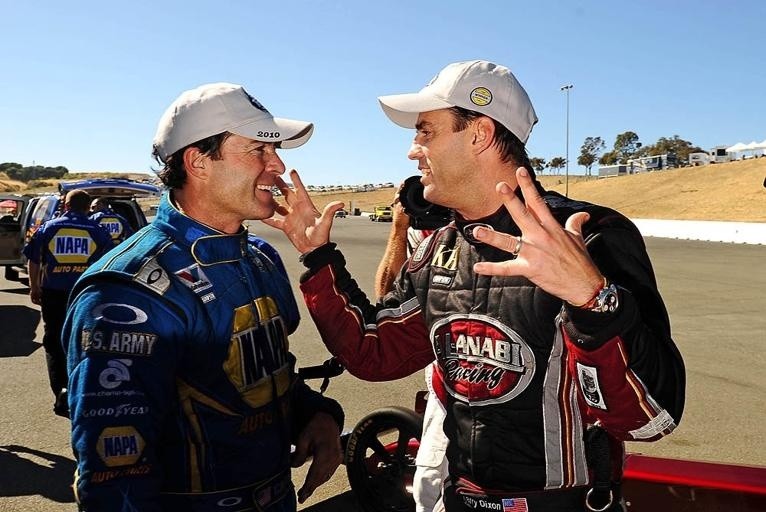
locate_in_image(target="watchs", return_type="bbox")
[582,278,619,313]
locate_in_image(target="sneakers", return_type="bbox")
[54,387,67,414]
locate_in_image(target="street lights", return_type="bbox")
[32,160,36,198]
[560,84,573,198]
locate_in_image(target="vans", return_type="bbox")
[0,177,161,276]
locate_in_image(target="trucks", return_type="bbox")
[369,206,393,222]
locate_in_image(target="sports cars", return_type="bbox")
[339,389,766,512]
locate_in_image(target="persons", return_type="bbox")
[260,60,686,512]
[22,190,112,415]
[61,84,346,512]
[89,198,134,245]
[374,176,456,511]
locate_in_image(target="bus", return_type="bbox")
[625,153,677,174]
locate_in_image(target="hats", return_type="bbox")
[151,83,315,163]
[378,60,539,148]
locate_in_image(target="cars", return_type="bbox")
[137,177,167,194]
[334,208,346,219]
[270,182,394,196]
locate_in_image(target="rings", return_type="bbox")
[514,236,522,257]
